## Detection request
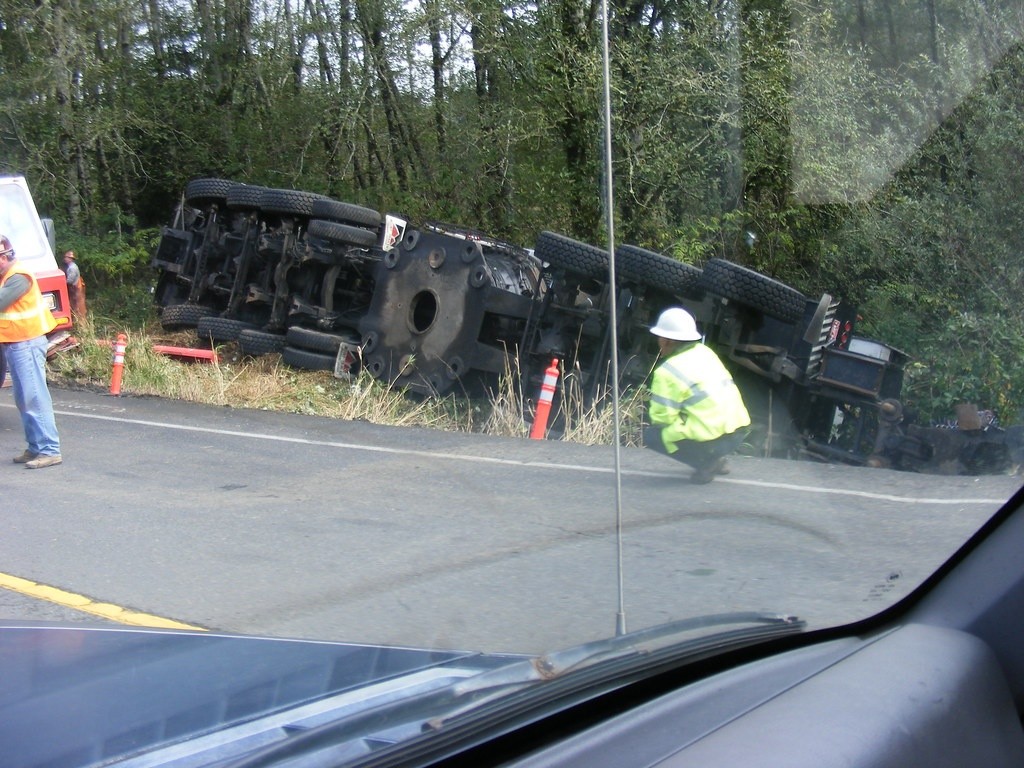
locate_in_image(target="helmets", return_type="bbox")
[650,308,702,341]
[0,235,13,254]
[64,251,76,260]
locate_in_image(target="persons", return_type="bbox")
[0,234,63,469]
[63,250,81,311]
[642,305,753,485]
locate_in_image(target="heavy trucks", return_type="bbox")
[149,178,1023,478]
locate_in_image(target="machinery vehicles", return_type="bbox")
[2,176,73,334]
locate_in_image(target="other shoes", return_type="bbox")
[716,458,731,475]
[14,449,39,463]
[25,453,63,469]
[690,456,726,485]
